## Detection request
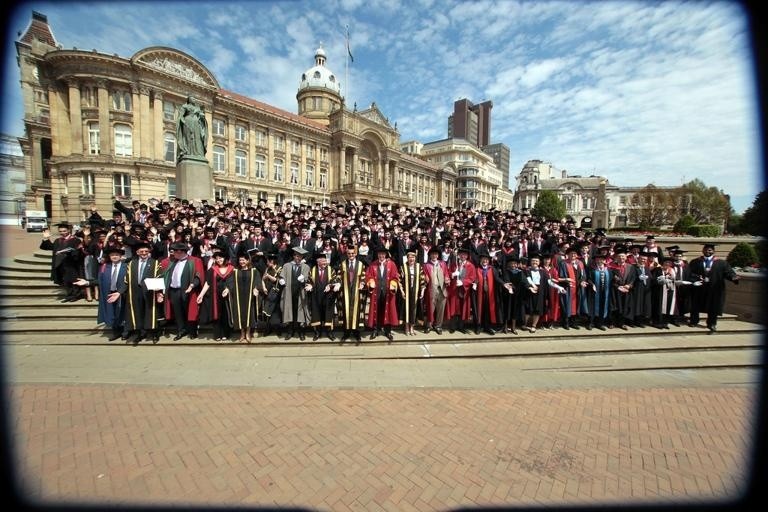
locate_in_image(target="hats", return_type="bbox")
[90,200,721,266]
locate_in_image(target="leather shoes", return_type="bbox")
[63,294,99,304]
[108,318,718,344]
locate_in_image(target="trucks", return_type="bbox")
[23,210,50,234]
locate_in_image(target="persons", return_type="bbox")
[176,96,209,159]
